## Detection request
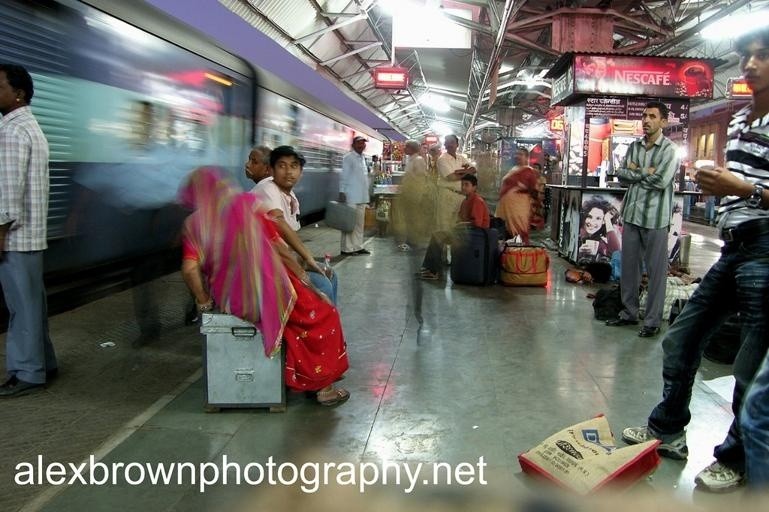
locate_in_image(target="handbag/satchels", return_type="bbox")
[667,289,691,327]
[583,261,613,284]
[499,234,550,287]
[364,207,377,228]
[517,412,663,509]
[636,276,701,322]
[592,285,623,321]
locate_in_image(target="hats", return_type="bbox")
[353,136,367,141]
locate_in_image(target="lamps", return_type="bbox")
[725,77,752,100]
[373,66,411,88]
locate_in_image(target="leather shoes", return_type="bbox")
[604,314,639,326]
[353,248,370,256]
[0,367,60,400]
[340,250,354,258]
[638,325,660,338]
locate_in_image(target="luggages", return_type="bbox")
[449,222,499,287]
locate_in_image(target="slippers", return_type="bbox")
[321,388,350,407]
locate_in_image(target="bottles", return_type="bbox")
[374,162,392,185]
[322,254,333,277]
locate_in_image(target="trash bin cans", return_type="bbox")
[680,234,691,264]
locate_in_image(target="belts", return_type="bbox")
[720,217,769,243]
[446,187,463,195]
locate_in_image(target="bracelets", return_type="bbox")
[194,297,214,313]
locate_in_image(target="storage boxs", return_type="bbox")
[199,309,288,413]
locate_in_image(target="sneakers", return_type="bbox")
[622,424,690,461]
[397,243,410,252]
[694,460,745,494]
[414,266,441,281]
[185,303,199,327]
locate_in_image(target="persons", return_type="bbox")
[529,161,546,231]
[173,163,350,411]
[243,142,275,182]
[618,24,769,492]
[412,173,490,279]
[436,134,477,196]
[581,195,621,259]
[339,136,371,256]
[604,102,679,336]
[574,56,597,93]
[0,63,62,399]
[248,144,338,311]
[63,95,199,350]
[739,342,769,492]
[388,139,428,253]
[417,141,442,183]
[673,165,717,228]
[493,146,540,248]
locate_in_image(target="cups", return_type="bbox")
[585,240,600,257]
[683,65,712,97]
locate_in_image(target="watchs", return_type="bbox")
[743,184,763,209]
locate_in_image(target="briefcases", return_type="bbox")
[325,200,357,234]
